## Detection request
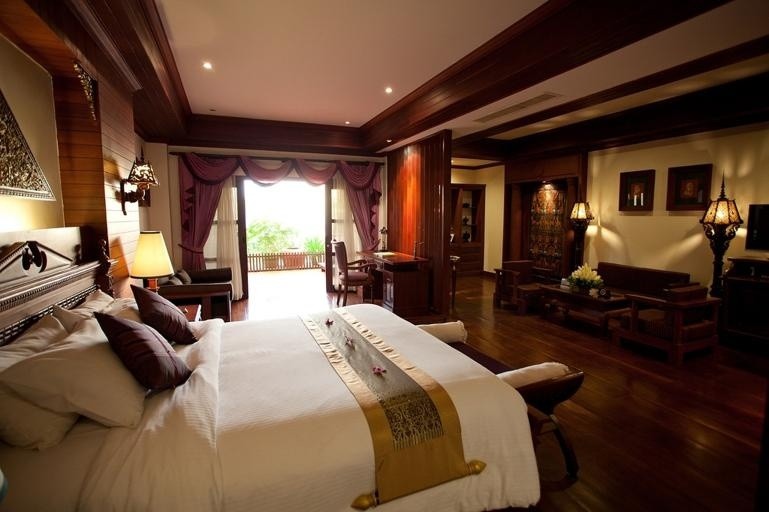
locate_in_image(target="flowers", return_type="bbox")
[569,261,604,287]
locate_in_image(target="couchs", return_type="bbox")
[159,266,234,320]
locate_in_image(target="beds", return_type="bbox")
[0,224,529,512]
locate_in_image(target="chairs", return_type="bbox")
[494,257,546,318]
[330,238,379,308]
[612,283,721,363]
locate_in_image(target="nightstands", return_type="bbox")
[175,302,204,322]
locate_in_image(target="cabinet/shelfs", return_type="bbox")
[450,182,486,278]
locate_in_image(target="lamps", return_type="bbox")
[567,183,596,266]
[117,158,158,215]
[697,169,745,300]
[126,227,175,291]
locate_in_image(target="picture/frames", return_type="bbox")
[618,162,714,213]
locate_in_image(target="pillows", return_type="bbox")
[0,281,208,455]
[168,269,192,286]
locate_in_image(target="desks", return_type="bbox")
[354,247,432,313]
[718,254,769,361]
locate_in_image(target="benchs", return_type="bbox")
[414,319,583,481]
[591,259,690,306]
